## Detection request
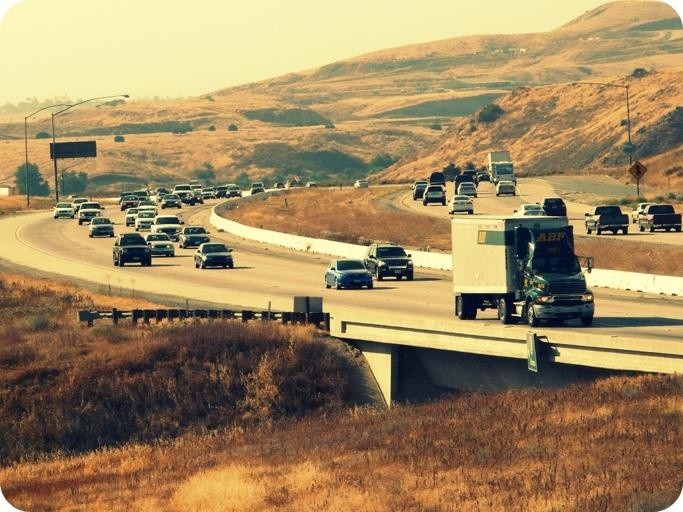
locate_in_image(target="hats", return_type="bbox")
[406,167,520,215]
[351,179,369,190]
[512,198,568,218]
[42,175,321,273]
[324,258,377,293]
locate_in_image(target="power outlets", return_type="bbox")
[582,203,682,233]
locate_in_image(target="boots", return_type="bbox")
[359,241,417,282]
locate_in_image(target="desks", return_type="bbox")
[51,91,134,206]
[569,79,635,168]
[23,100,77,211]
